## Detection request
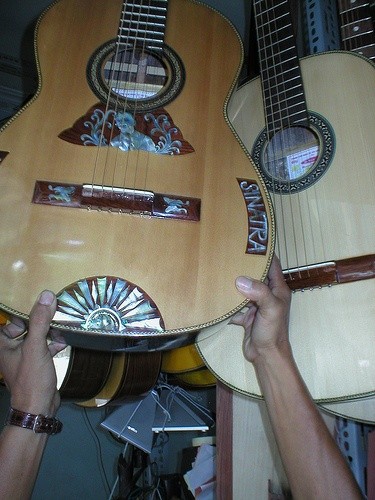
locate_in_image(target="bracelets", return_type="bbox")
[3,408,63,435]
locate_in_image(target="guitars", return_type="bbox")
[195,0,375,428]
[0,0,276,410]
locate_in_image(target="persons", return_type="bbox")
[0,251,367,500]
